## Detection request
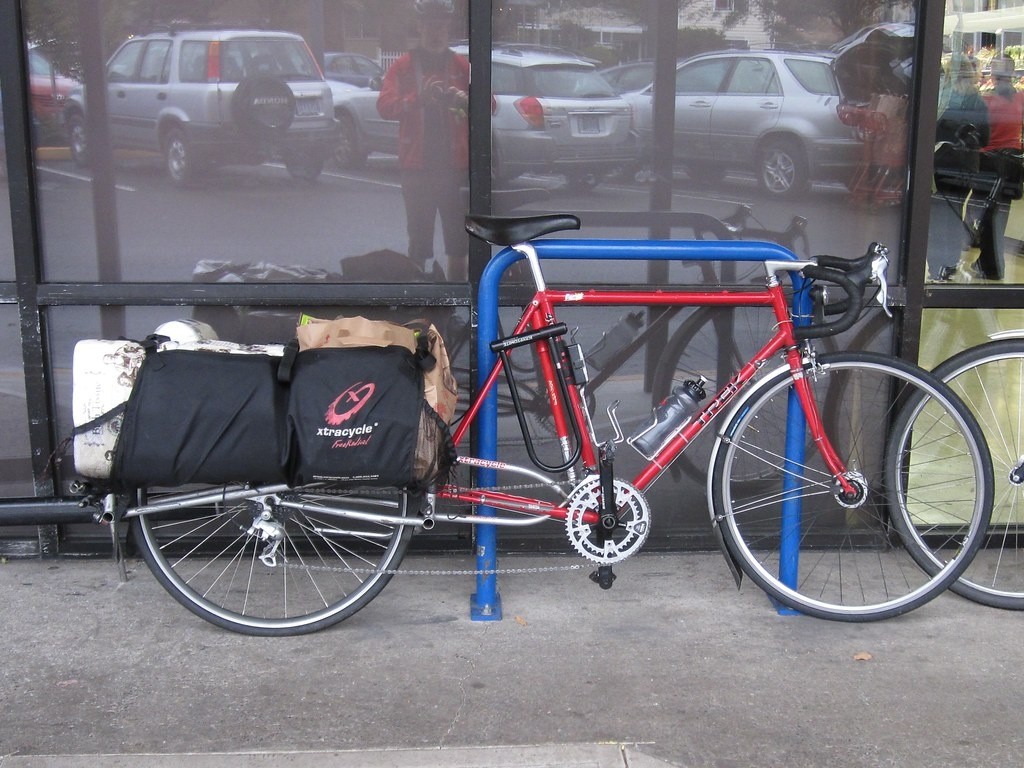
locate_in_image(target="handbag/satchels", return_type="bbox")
[72,334,457,565]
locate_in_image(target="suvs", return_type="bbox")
[65,30,343,190]
[323,41,635,189]
[598,23,917,201]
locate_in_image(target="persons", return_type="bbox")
[933,49,1024,281]
[835,54,915,239]
[377,0,496,284]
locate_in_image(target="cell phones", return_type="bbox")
[434,91,455,105]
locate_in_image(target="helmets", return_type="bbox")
[414,0,456,19]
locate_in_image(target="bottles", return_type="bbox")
[630,375,708,458]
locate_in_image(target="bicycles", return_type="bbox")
[43,214,1024,639]
[192,205,842,498]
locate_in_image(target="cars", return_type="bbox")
[29,54,83,149]
